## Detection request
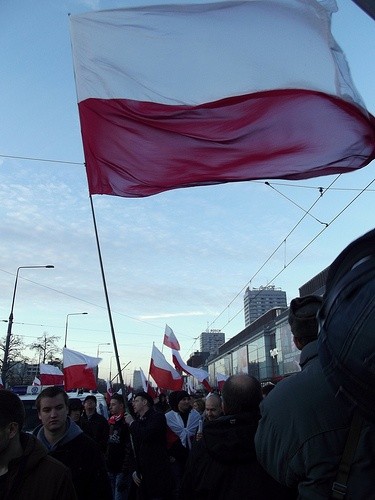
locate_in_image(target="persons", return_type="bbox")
[0,230,375,500]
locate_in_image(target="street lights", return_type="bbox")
[0,265,55,389]
[97,342,111,357]
[109,356,120,381]
[64,312,88,348]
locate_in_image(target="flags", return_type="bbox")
[39,364,63,385]
[171,349,210,381]
[217,373,229,392]
[140,370,158,401]
[69,0,375,198]
[198,379,213,392]
[150,345,182,390]
[63,346,101,391]
[106,379,113,404]
[163,324,180,350]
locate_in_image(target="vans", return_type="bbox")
[11,385,109,436]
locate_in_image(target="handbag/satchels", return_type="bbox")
[317,227,375,416]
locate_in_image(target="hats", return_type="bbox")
[85,395,97,404]
[288,294,323,338]
